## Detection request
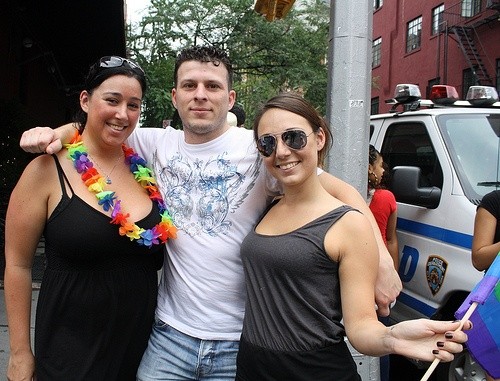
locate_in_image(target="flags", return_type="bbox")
[454,251,500,381]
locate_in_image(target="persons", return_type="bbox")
[234,95,473,381]
[472,190,500,276]
[20,45,403,381]
[4,56,179,381]
[170,106,245,130]
[367,144,399,381]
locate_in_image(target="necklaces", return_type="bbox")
[65,131,178,248]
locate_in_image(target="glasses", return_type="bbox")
[256,129,314,157]
[97,55,145,77]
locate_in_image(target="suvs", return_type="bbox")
[365,83,500,381]
